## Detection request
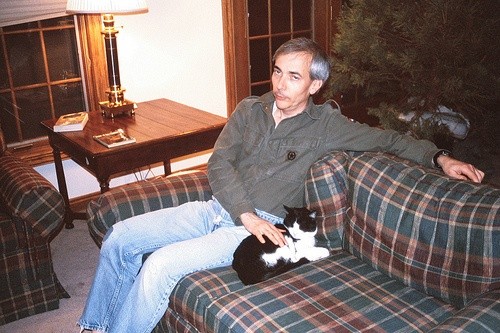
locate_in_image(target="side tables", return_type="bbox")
[41,99,228,229]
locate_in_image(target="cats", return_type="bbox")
[231,204,330,286]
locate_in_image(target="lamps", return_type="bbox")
[66,1,148,119]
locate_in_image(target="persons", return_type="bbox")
[76,37,485,333]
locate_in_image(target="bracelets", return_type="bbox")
[434,150,451,168]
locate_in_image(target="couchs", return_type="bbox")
[86,151,500,333]
[0,130,71,325]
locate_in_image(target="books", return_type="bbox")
[53,112,89,132]
[93,129,136,147]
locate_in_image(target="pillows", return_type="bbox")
[301,157,351,250]
